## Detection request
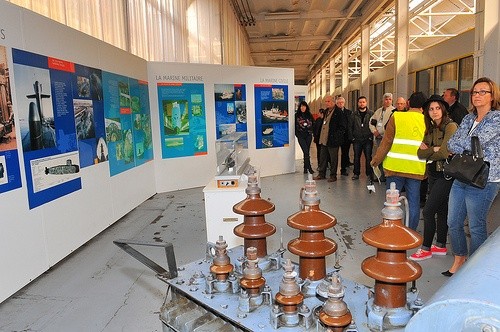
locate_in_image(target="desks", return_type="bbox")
[202,166,250,254]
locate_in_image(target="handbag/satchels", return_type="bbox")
[444,135,490,189]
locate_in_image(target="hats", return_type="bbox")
[427,93,450,111]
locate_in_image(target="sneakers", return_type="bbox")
[431,245,448,255]
[409,248,433,260]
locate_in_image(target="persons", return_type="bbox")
[351,96,374,180]
[295,101,315,174]
[368,92,429,230]
[312,96,352,182]
[443,88,469,125]
[410,95,459,261]
[442,77,500,277]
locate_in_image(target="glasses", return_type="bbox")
[469,90,491,95]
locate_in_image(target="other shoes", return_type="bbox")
[352,175,359,180]
[313,174,322,180]
[304,169,308,174]
[441,269,453,277]
[309,168,314,174]
[328,176,337,182]
